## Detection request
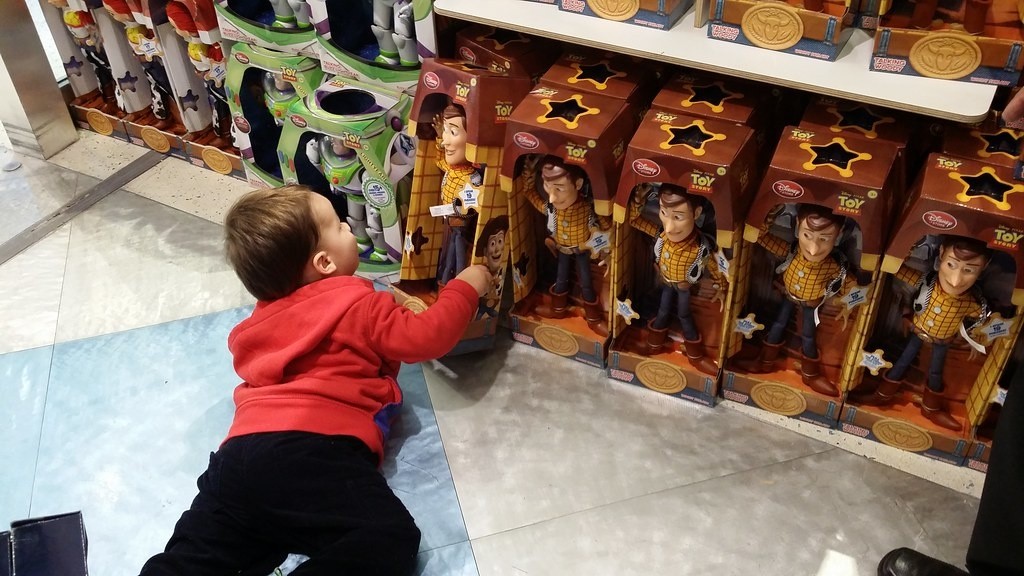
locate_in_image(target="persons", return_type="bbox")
[270,0,310,32]
[428,103,481,300]
[49,1,237,149]
[860,238,994,432]
[302,134,390,261]
[139,184,492,576]
[735,207,860,394]
[263,74,300,131]
[627,183,727,376]
[521,152,613,338]
[371,1,419,66]
[878,360,1024,576]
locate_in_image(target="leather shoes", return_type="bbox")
[877,547,969,576]
